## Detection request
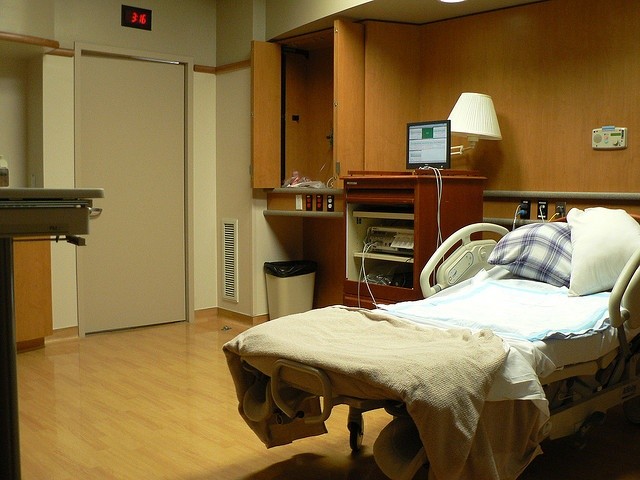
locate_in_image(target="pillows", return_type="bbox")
[488,223,573,287]
[568,207,640,296]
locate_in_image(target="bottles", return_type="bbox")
[0,155,11,189]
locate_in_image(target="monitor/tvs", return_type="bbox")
[406,120,451,169]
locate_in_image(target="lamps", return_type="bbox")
[447,93,503,155]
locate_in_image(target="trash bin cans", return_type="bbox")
[264,260,317,320]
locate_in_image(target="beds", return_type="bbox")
[224,209,640,480]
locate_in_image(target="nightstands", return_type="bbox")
[342,169,486,307]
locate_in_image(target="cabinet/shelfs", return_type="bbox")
[250,17,365,217]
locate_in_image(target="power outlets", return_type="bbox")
[554,202,567,218]
[520,201,529,218]
[537,200,548,219]
